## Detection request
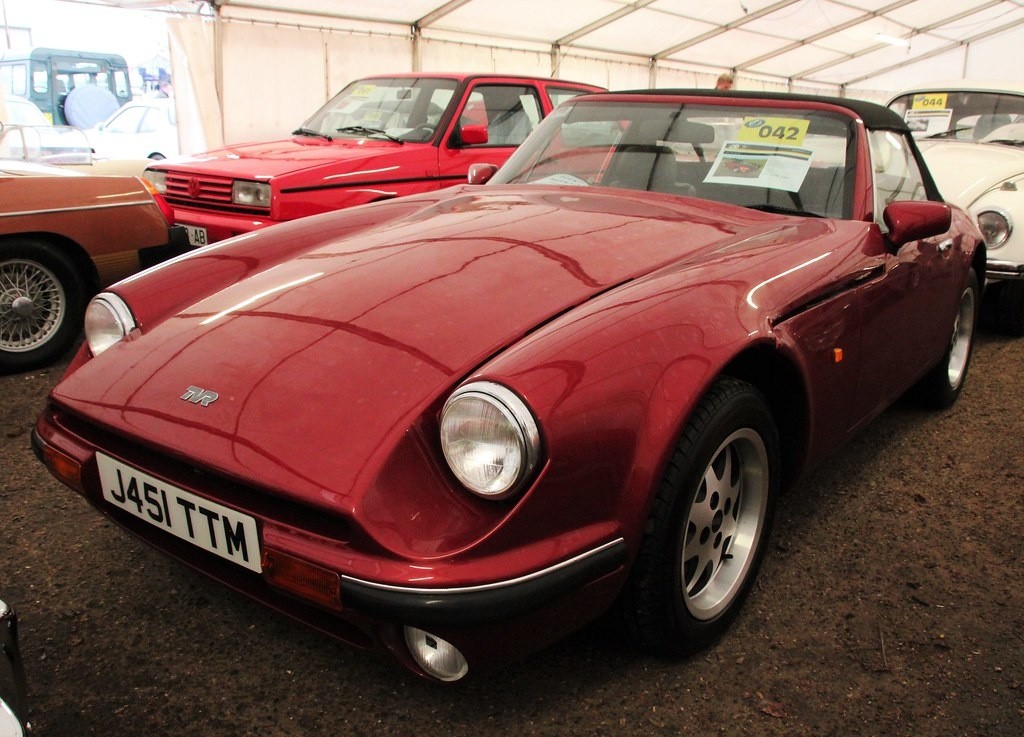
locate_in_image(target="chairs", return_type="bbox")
[485,111,531,145]
[610,145,696,200]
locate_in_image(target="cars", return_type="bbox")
[140,73,1023,338]
[0,95,180,376]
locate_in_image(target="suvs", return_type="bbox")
[0,47,132,131]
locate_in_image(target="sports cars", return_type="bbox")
[31,87,986,686]
